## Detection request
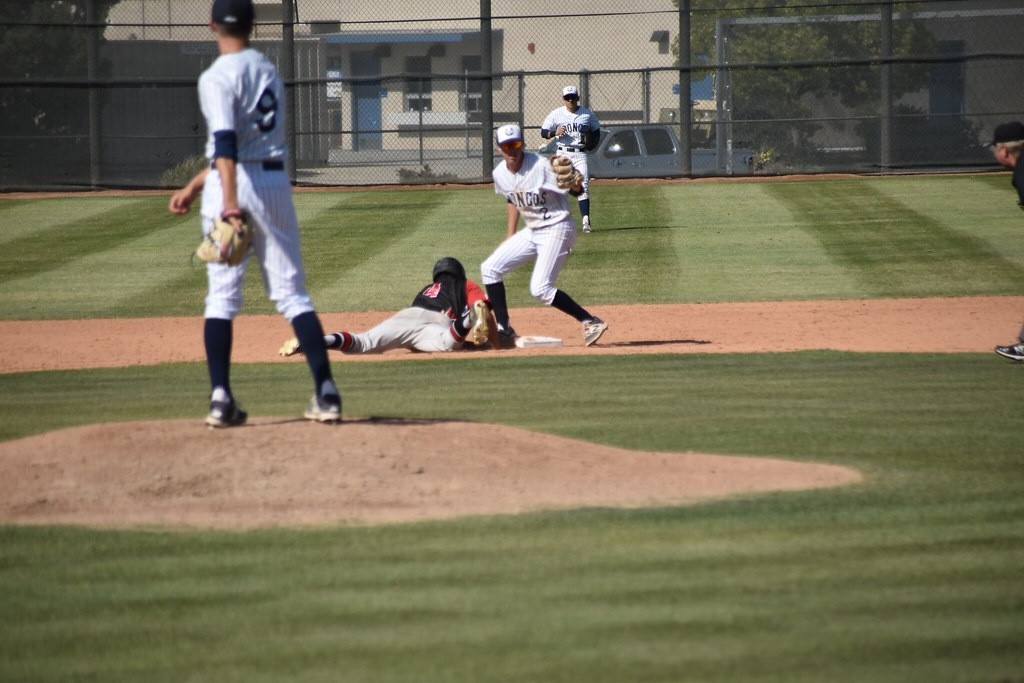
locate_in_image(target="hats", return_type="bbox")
[213,0,257,26]
[496,125,524,144]
[983,121,1024,148]
[562,85,578,97]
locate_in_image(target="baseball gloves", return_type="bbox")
[549,154,585,190]
[188,208,256,276]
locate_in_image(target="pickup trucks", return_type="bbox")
[539,123,755,177]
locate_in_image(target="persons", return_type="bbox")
[480,125,608,349]
[981,122,1024,362]
[279,257,495,357]
[541,87,601,233]
[170,0,342,428]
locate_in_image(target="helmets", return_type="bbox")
[432,257,465,282]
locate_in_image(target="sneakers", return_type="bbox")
[995,337,1024,361]
[581,222,592,233]
[583,316,609,349]
[204,400,248,428]
[497,327,517,342]
[469,300,490,346]
[278,336,302,357]
[302,392,343,421]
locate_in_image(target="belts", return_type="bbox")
[558,147,585,153]
[210,159,284,172]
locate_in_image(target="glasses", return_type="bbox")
[499,141,524,149]
[564,94,578,101]
